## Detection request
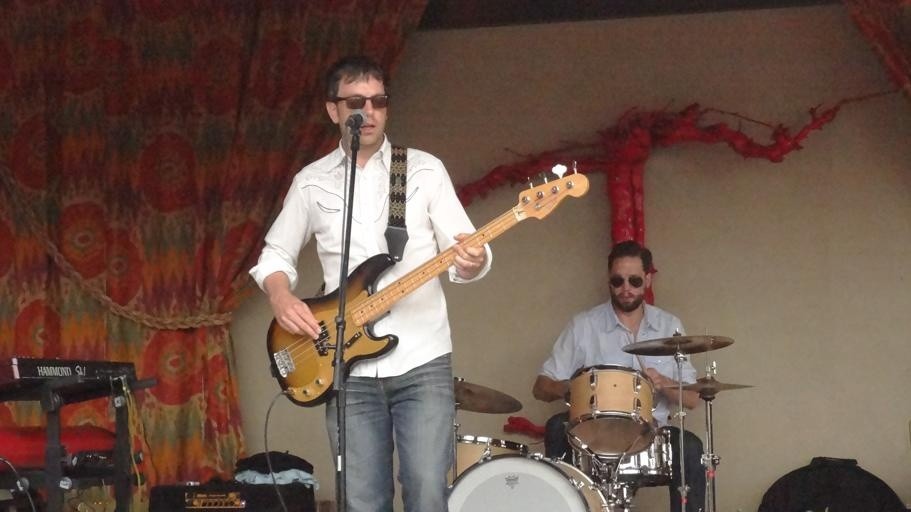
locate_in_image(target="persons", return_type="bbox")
[532,240,707,512]
[246,55,493,512]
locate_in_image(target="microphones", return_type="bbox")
[344,111,368,130]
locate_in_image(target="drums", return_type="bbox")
[448,453,611,511]
[563,364,655,458]
[446,434,527,494]
[572,427,672,486]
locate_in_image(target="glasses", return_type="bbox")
[607,273,646,288]
[327,91,390,111]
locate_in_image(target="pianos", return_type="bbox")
[0,359,136,386]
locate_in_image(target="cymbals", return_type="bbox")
[622,334,734,354]
[453,380,523,414]
[663,378,753,400]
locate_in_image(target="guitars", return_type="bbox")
[266,160,590,406]
